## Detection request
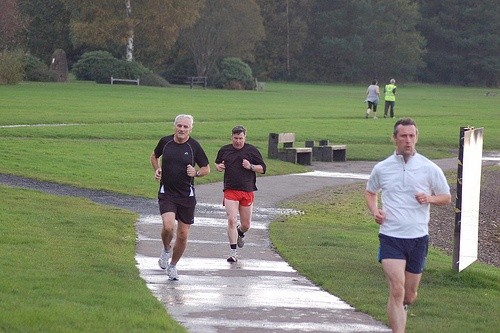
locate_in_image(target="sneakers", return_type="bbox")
[159,247,171,270]
[227,255,237,262]
[236,225,245,248]
[167,266,179,281]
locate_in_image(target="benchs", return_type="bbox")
[268,132,313,165]
[305,140,346,163]
[111,77,140,86]
[186,77,207,90]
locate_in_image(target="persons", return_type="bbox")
[151,114,210,281]
[365,79,380,119]
[384,79,397,119]
[215,126,266,262]
[365,119,452,333]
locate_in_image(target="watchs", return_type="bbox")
[196,170,200,177]
[250,164,254,171]
